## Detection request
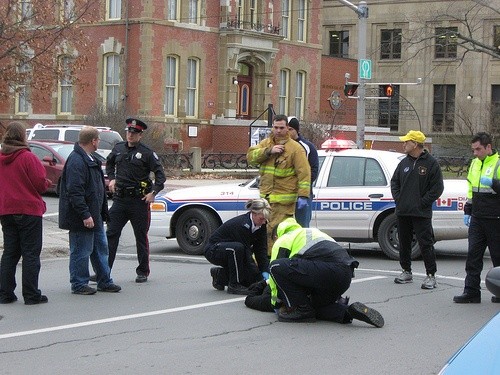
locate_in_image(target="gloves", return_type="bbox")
[464,214,471,227]
[297,198,307,209]
[480,177,492,187]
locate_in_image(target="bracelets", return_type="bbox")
[152,190,156,195]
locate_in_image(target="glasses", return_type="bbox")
[91,138,101,142]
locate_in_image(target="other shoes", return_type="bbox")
[228,283,249,294]
[454,292,481,303]
[211,267,224,291]
[39,295,48,304]
[492,295,500,302]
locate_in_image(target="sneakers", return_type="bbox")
[98,284,121,292]
[350,301,384,327]
[422,276,436,289]
[394,269,413,284]
[279,306,315,322]
[72,285,96,295]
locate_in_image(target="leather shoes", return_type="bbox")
[89,275,98,282]
[136,275,146,282]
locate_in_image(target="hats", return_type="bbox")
[399,130,426,144]
[287,116,300,131]
[124,117,147,133]
[278,218,297,236]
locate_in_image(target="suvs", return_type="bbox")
[25,123,124,168]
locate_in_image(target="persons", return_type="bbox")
[202,198,272,295]
[286,117,318,226]
[391,130,444,289]
[89,118,166,283]
[0,122,52,304]
[453,132,500,304]
[268,218,384,327]
[59,127,121,295]
[246,114,311,262]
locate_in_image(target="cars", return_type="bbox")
[437,268,500,375]
[27,140,117,198]
[147,140,473,261]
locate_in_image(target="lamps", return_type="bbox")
[267,81,273,88]
[466,93,474,100]
[232,77,239,85]
[119,91,129,102]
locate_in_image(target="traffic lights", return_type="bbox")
[344,84,360,97]
[384,85,395,97]
